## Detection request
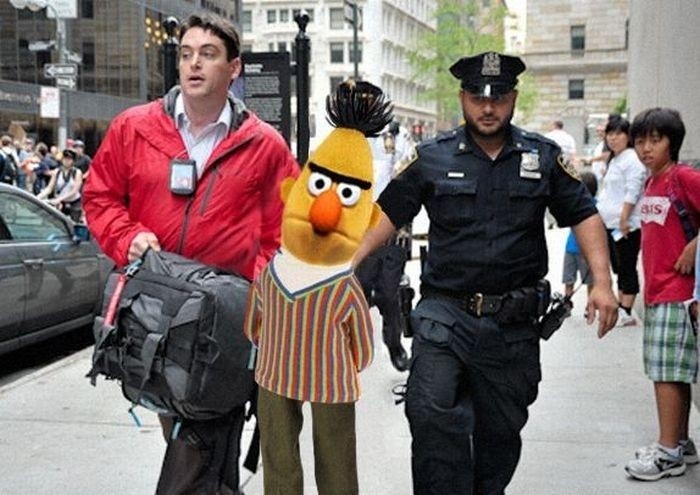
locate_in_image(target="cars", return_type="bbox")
[0,182,115,360]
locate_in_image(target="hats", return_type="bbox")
[448,49,527,101]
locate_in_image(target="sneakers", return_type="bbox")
[624,438,697,482]
[616,307,638,328]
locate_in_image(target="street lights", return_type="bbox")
[26,1,69,156]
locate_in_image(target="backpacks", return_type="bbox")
[85,247,256,424]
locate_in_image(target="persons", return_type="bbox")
[353,122,418,375]
[595,115,650,326]
[543,120,576,229]
[351,52,617,495]
[580,124,605,180]
[623,109,700,483]
[562,173,600,318]
[0,134,93,223]
[81,12,303,495]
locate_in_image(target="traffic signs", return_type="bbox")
[55,75,78,92]
[342,1,363,31]
[44,62,79,79]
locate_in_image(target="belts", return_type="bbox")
[431,291,503,316]
